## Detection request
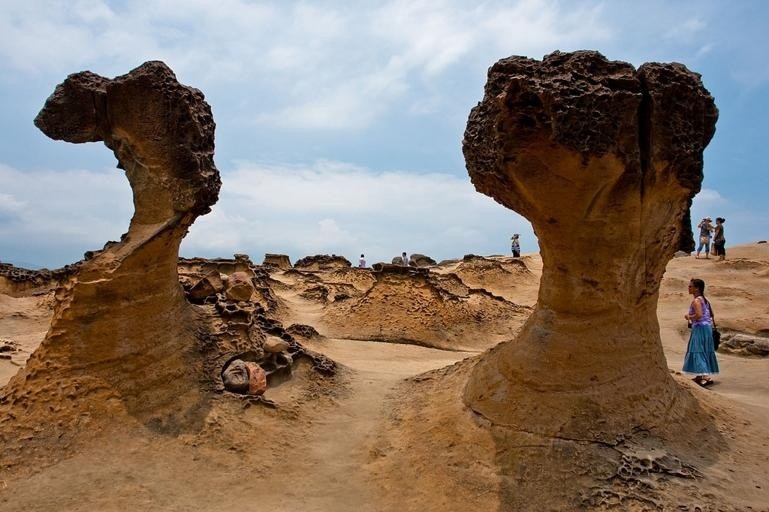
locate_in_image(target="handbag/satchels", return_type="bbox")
[702,236,707,243]
[711,243,717,256]
[712,330,720,350]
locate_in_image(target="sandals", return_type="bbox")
[700,378,713,387]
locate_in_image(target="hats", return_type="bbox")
[703,217,712,222]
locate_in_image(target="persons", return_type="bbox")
[696,216,715,259]
[511,234,521,257]
[713,218,726,261]
[402,252,408,266]
[682,279,719,387]
[359,254,366,267]
